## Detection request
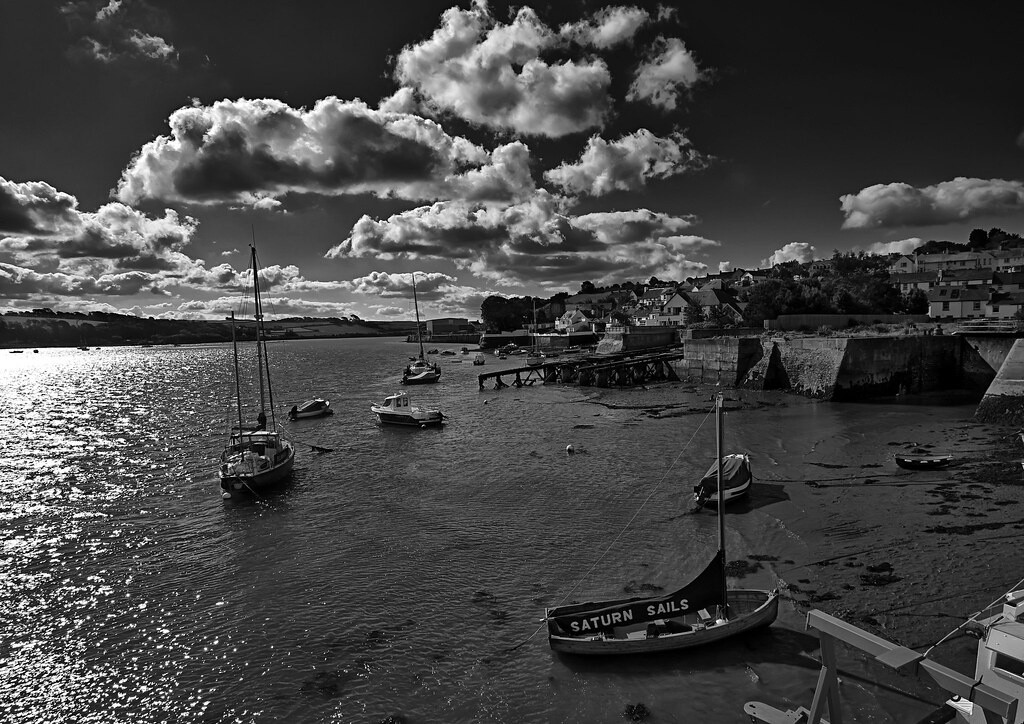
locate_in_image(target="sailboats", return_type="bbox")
[543,389,781,656]
[217,240,298,496]
[401,271,444,386]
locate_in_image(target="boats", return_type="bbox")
[369,391,446,428]
[427,299,583,368]
[288,395,331,423]
[892,453,955,469]
[690,451,754,503]
